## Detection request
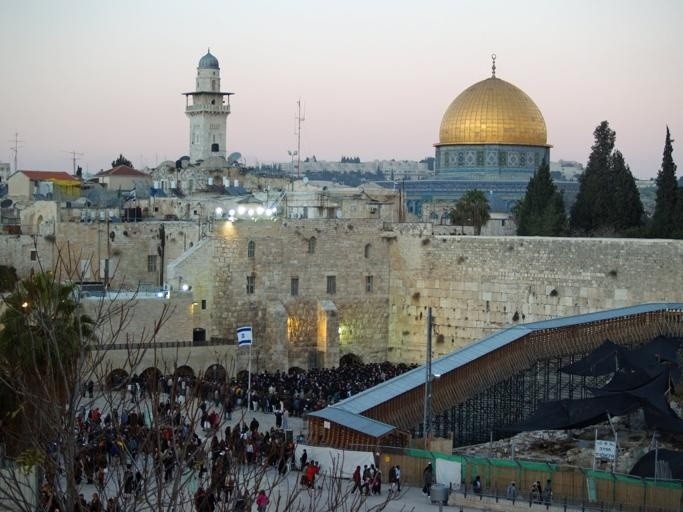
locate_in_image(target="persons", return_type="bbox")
[350,464,400,495]
[545,480,552,508]
[82,378,93,398]
[507,481,516,504]
[537,481,543,504]
[529,483,540,508]
[127,371,197,402]
[421,462,433,498]
[40,403,307,511]
[197,360,421,431]
[474,476,481,493]
[303,459,321,488]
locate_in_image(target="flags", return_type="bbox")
[236,326,252,347]
